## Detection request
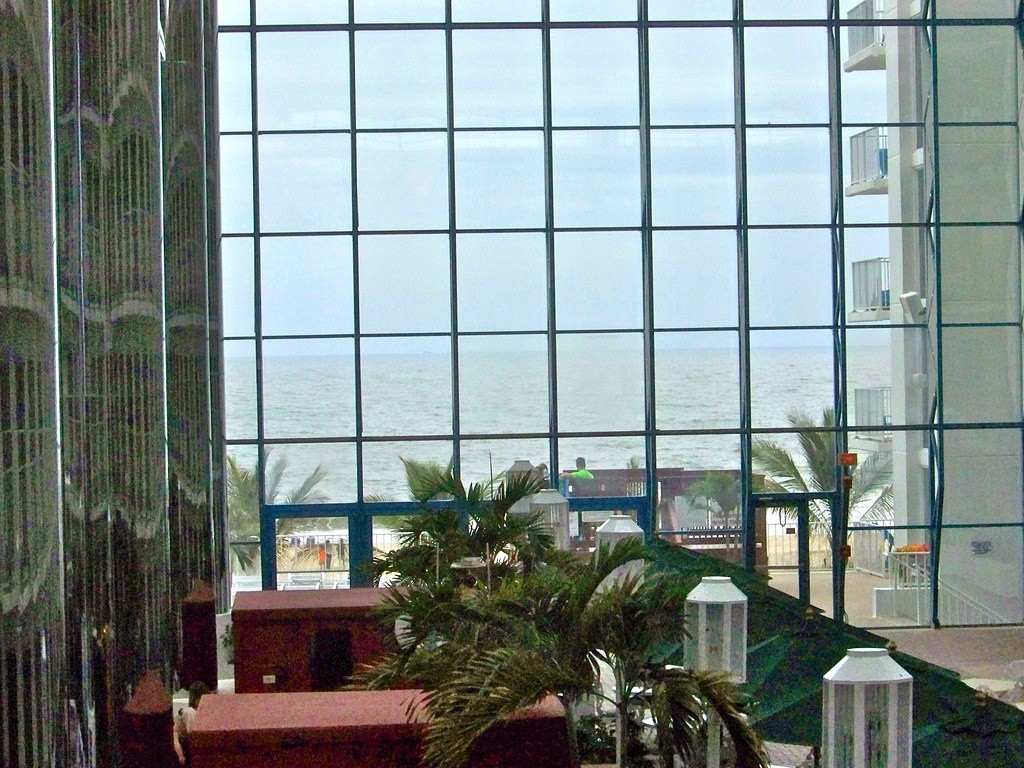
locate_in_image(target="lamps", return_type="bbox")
[529,489,570,552]
[821,648,913,768]
[595,516,644,763]
[837,453,856,489]
[506,460,537,517]
[682,576,748,768]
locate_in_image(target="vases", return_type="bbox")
[119,706,181,768]
[180,600,217,691]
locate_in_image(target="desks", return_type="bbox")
[190,689,569,768]
[231,586,419,694]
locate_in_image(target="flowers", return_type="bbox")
[179,577,214,602]
[897,544,931,552]
[125,667,170,713]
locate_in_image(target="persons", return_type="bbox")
[319,540,332,570]
[560,457,594,479]
[174,681,209,764]
[336,538,347,565]
